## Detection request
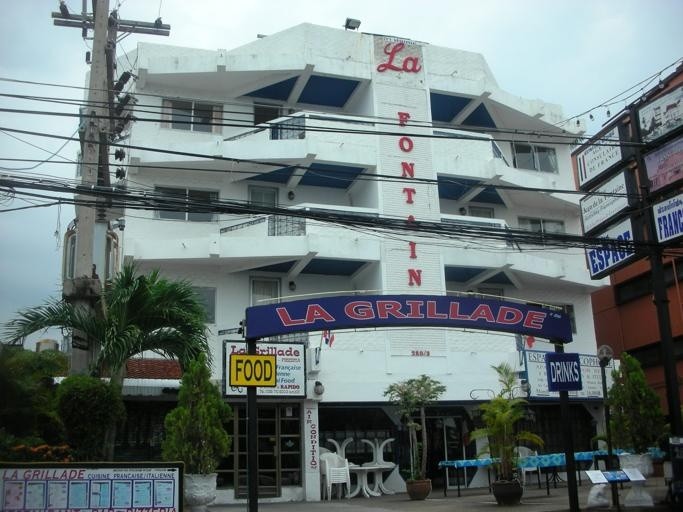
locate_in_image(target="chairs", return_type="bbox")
[320,453,350,502]
[514,446,541,490]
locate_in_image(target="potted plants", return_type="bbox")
[589,352,667,479]
[163,351,235,508]
[471,362,545,507]
[383,374,446,500]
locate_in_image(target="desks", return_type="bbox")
[346,466,396,498]
[439,447,666,498]
